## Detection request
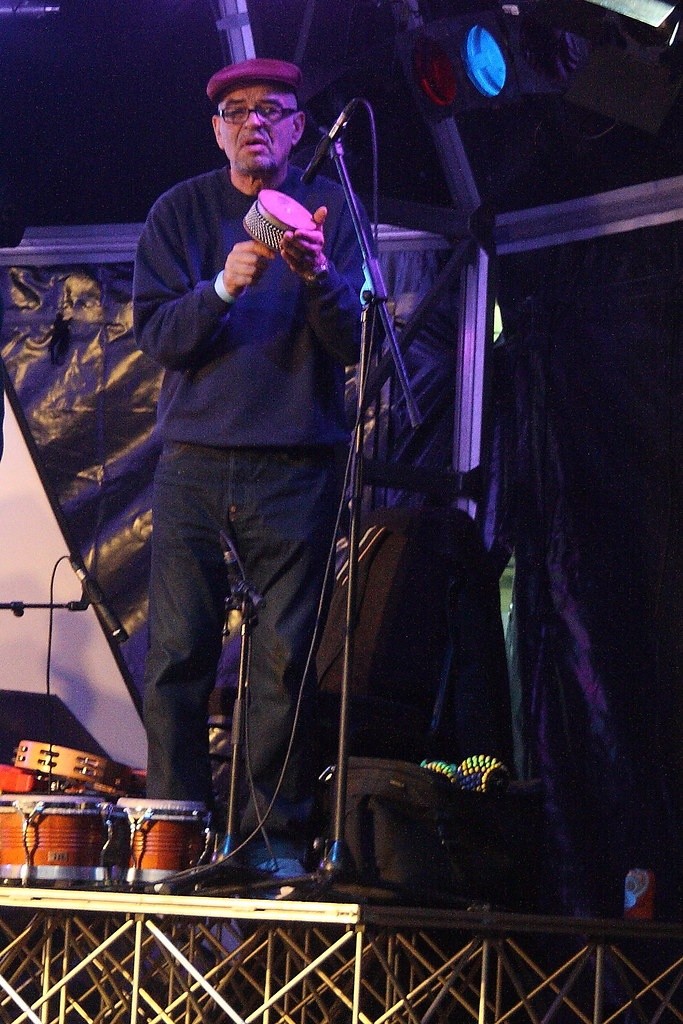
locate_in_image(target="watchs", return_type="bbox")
[306,256,330,284]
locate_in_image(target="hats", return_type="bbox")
[206,59,304,103]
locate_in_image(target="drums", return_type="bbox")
[0,792,212,888]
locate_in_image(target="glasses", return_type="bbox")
[215,103,299,125]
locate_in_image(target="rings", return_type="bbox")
[303,255,310,264]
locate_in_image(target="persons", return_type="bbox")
[134,58,378,900]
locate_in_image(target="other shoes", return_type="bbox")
[253,858,308,901]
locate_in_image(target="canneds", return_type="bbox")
[623,868,655,920]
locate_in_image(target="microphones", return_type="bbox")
[70,559,131,645]
[299,97,359,186]
[220,522,247,611]
